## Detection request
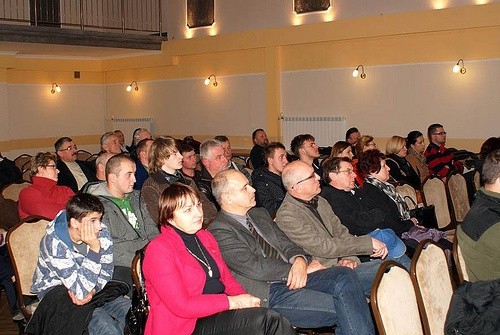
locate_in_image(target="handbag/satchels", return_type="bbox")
[384,158,410,188]
[404,196,438,230]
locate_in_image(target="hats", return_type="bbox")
[369,228,406,258]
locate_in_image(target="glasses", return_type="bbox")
[366,143,377,147]
[292,171,316,189]
[58,145,78,152]
[37,164,57,170]
[434,131,446,136]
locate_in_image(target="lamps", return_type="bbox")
[50,83,62,94]
[127,79,139,93]
[352,65,366,79]
[204,74,218,88]
[453,59,467,76]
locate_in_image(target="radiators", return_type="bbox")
[114,116,152,146]
[280,112,350,151]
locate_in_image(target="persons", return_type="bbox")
[0,124,500,335]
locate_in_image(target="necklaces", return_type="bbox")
[185,237,213,277]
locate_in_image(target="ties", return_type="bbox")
[246,217,284,261]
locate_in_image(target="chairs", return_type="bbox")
[0,150,474,335]
